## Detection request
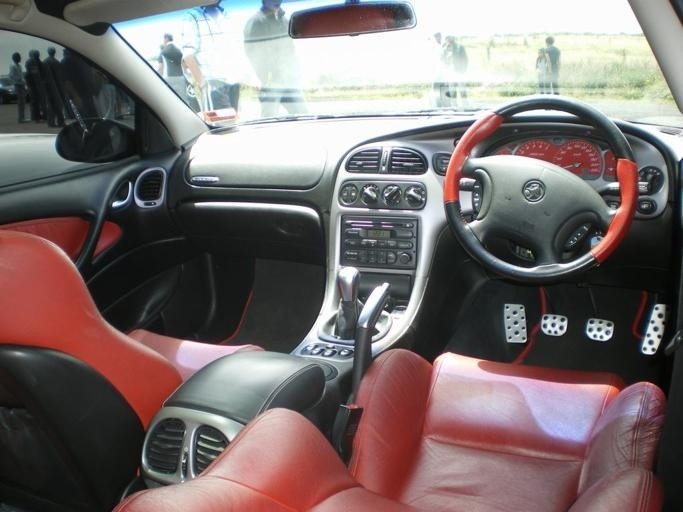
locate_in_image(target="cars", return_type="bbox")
[0,73,31,104]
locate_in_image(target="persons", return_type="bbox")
[243,1,303,117]
[429,32,560,108]
[178,1,238,115]
[8,34,182,128]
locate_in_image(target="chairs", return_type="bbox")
[111,346,666,512]
[0,229,266,511]
